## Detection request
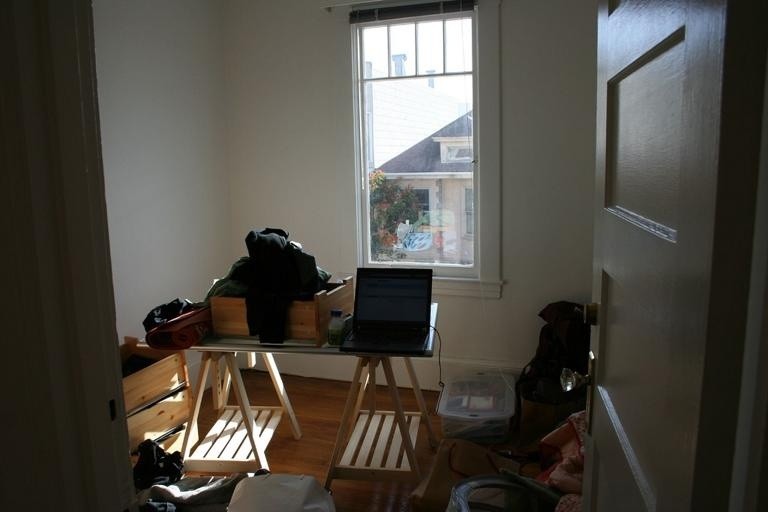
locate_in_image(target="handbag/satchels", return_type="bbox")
[422,438,521,508]
[225,474,334,512]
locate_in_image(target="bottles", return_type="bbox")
[328,310,346,346]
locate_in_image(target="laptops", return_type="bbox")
[339,267,434,355]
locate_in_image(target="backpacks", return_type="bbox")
[519,301,589,405]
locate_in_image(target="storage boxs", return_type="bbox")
[437,371,515,439]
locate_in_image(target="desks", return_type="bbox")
[136,301,439,495]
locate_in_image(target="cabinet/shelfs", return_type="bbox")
[119,335,200,470]
